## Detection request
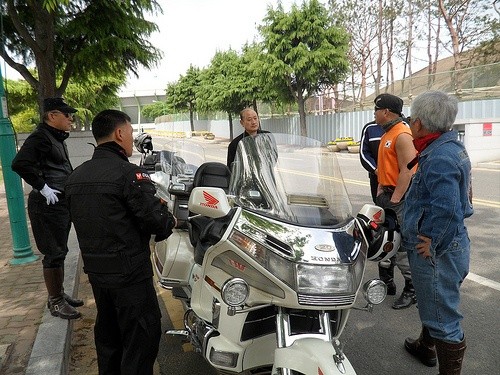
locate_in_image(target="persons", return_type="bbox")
[11,96,84,319]
[402,90,474,375]
[359,92,418,310]
[67,110,176,375]
[227,108,279,194]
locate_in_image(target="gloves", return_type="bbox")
[39,183,62,205]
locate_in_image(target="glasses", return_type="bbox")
[406,116,412,122]
[375,107,382,111]
[52,112,71,118]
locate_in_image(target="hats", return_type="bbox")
[374,94,403,113]
[44,98,77,113]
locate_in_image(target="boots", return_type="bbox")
[435,333,467,375]
[378,263,396,295]
[392,278,417,309]
[43,267,81,319]
[63,288,84,307]
[406,327,437,367]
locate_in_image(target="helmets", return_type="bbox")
[367,208,402,262]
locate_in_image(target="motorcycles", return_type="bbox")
[138,132,387,375]
